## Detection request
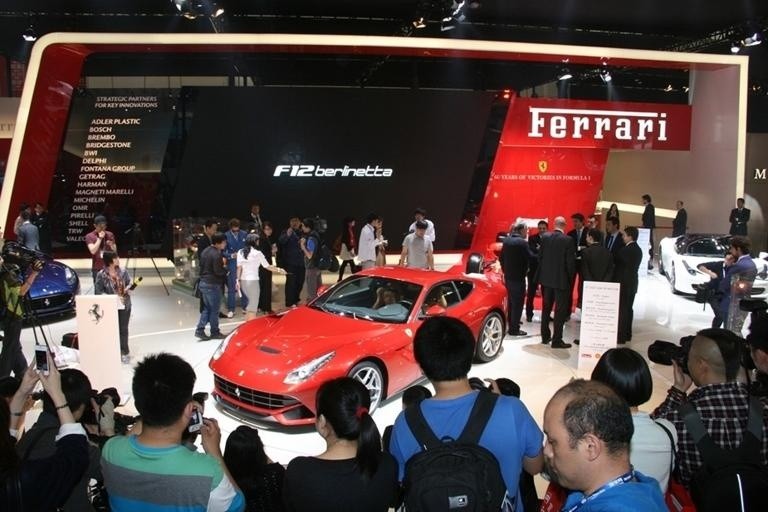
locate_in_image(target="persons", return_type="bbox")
[0,314,767,512]
[500,194,768,348]
[1,204,131,363]
[194,195,438,341]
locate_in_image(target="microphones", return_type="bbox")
[130,277,143,290]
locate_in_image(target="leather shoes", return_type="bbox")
[511,316,578,348]
[219,312,227,318]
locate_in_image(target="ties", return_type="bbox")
[608,236,614,249]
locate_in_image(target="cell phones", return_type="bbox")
[35,345,49,375]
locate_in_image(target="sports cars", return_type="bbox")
[658,232,768,299]
[209,266,510,434]
[21,259,79,321]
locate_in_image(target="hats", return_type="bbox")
[43,368,98,403]
[94,216,107,224]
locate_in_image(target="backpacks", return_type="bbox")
[403,388,515,512]
[678,393,768,512]
[334,234,343,253]
[310,234,333,270]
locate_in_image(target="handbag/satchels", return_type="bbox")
[655,421,696,512]
[376,247,385,266]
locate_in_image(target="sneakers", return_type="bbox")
[210,332,226,339]
[195,330,209,340]
[227,311,233,318]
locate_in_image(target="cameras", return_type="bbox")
[469,377,485,389]
[737,335,756,370]
[188,409,204,433]
[400,230,414,239]
[647,335,695,374]
[79,387,120,425]
[132,221,141,234]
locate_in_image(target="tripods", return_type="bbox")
[125,234,170,295]
[0,272,57,369]
